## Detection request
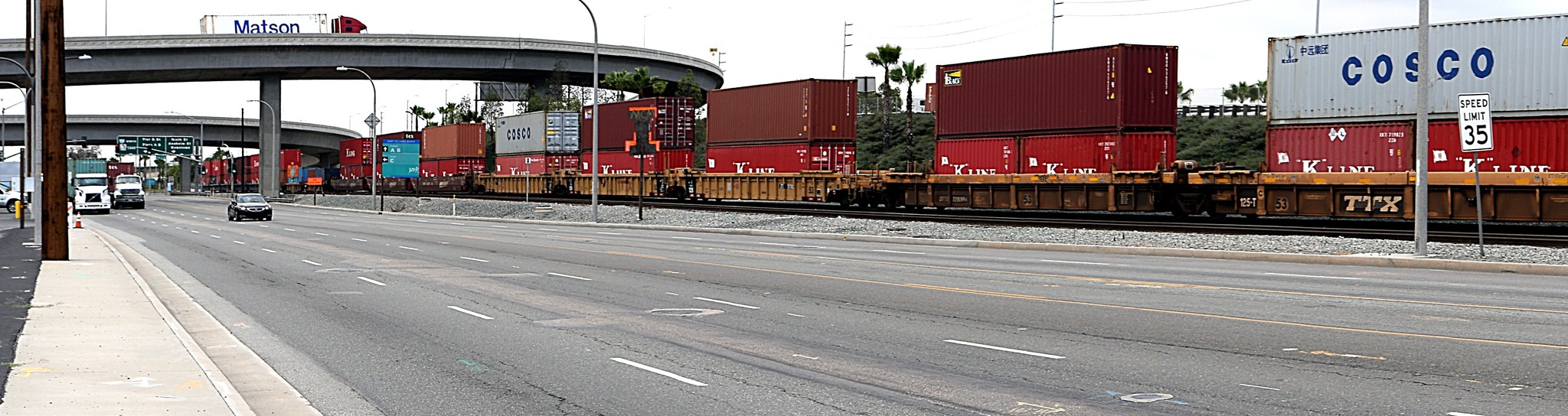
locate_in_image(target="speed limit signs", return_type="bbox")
[1458,92,1494,153]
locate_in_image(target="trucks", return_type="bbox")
[106,160,145,210]
[66,157,113,214]
[200,14,368,35]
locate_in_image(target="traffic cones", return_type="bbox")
[72,211,84,228]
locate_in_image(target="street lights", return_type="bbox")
[163,111,203,191]
[407,95,419,131]
[350,113,360,130]
[446,84,459,108]
[0,54,93,220]
[336,65,376,210]
[246,100,275,204]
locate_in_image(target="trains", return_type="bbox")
[199,11,1567,224]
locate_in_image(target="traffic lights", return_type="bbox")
[198,167,201,175]
[147,145,152,155]
[115,144,119,153]
[232,164,236,173]
[201,166,206,175]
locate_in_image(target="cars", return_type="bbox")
[228,193,273,222]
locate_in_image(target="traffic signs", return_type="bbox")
[165,136,194,156]
[382,139,420,178]
[137,135,167,156]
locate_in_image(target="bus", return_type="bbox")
[0,183,28,213]
